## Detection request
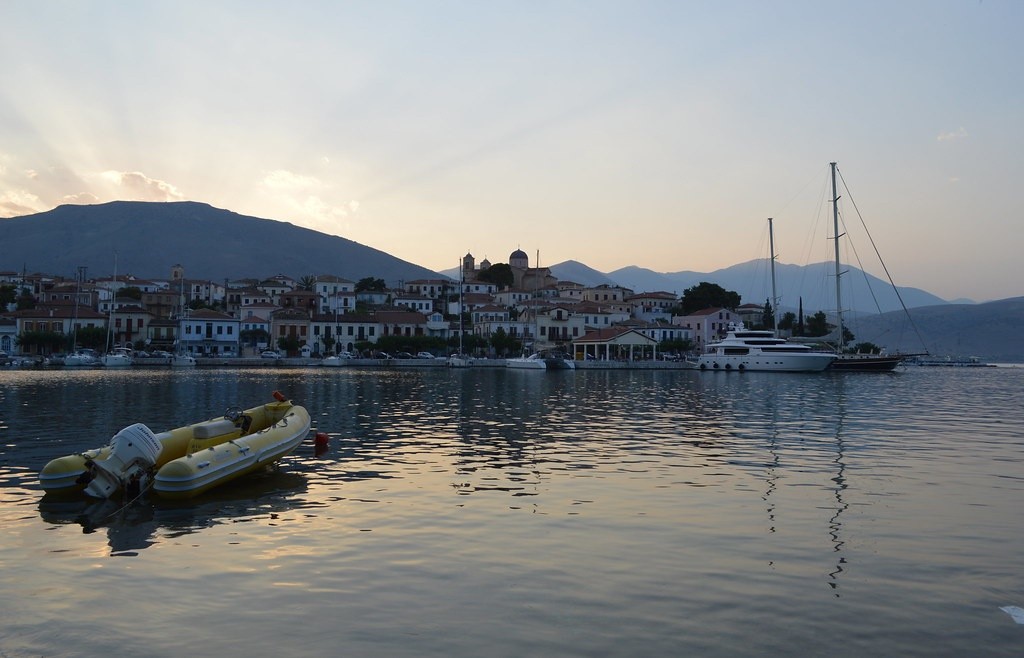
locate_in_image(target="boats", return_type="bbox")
[697,322,839,372]
[38,391,328,517]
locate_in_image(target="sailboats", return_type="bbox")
[748,161,931,373]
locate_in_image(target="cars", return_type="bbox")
[0,348,479,365]
